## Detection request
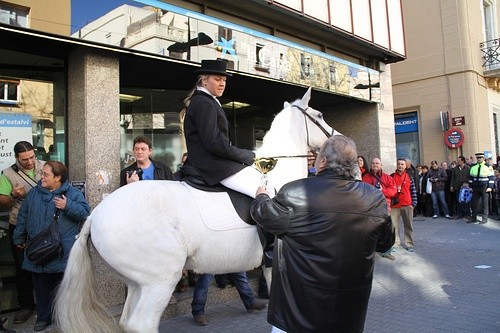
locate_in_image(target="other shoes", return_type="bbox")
[34,320,46,330]
[466,215,471,219]
[387,255,395,260]
[407,247,415,252]
[445,214,453,219]
[456,215,463,219]
[466,216,477,223]
[432,215,439,219]
[426,179,432,194]
[14,309,33,323]
[479,217,487,223]
[392,247,398,251]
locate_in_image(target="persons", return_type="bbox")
[169,270,267,326]
[180,62,277,268]
[120,135,176,187]
[390,158,417,252]
[357,156,368,176]
[363,158,397,260]
[410,156,500,219]
[250,135,396,333]
[306,151,317,176]
[465,153,494,224]
[12,160,90,331]
[174,153,188,181]
[0,141,46,322]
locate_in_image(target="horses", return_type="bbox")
[50,86,346,333]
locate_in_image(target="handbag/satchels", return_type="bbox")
[26,220,64,267]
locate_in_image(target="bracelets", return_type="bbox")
[10,193,17,199]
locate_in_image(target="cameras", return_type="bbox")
[128,168,143,177]
[375,184,380,189]
[397,185,401,193]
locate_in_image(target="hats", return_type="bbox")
[194,60,232,76]
[475,153,485,158]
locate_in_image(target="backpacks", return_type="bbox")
[459,186,473,204]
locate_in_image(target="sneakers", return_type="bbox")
[246,302,266,311]
[194,314,208,325]
